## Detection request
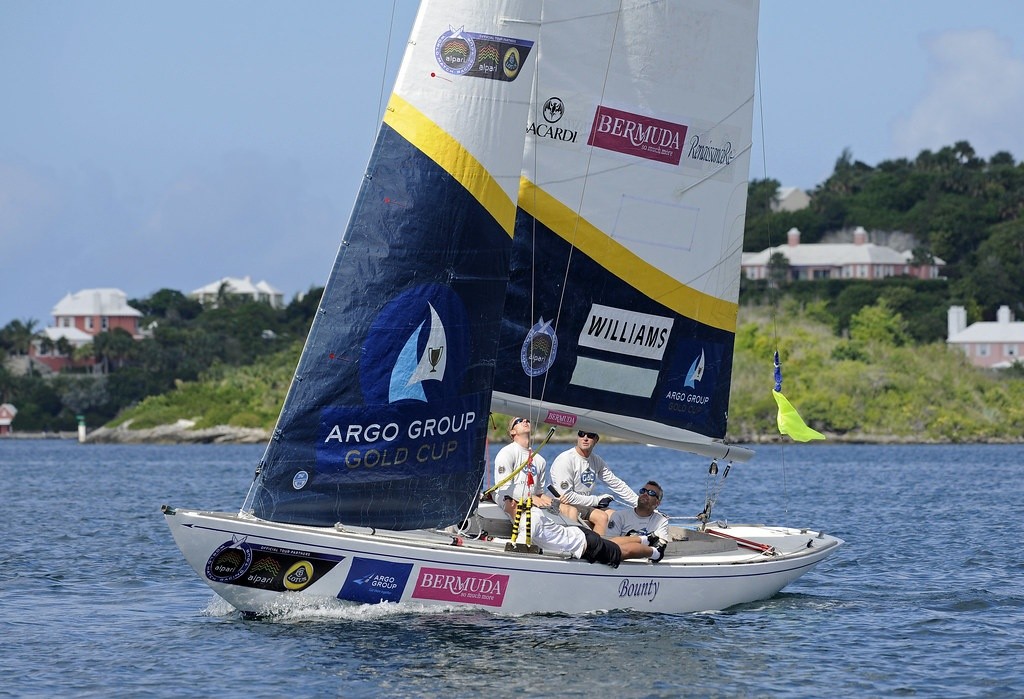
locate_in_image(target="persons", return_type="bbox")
[490,416,579,525]
[497,481,668,570]
[549,430,668,538]
[605,481,673,552]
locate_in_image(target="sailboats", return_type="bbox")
[156,0,846,627]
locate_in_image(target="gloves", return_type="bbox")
[598,493,614,506]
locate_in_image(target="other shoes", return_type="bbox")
[647,535,659,545]
[650,545,666,562]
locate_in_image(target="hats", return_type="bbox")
[497,483,529,502]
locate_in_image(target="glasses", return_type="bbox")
[639,489,659,499]
[503,496,511,500]
[511,419,530,430]
[578,430,596,438]
